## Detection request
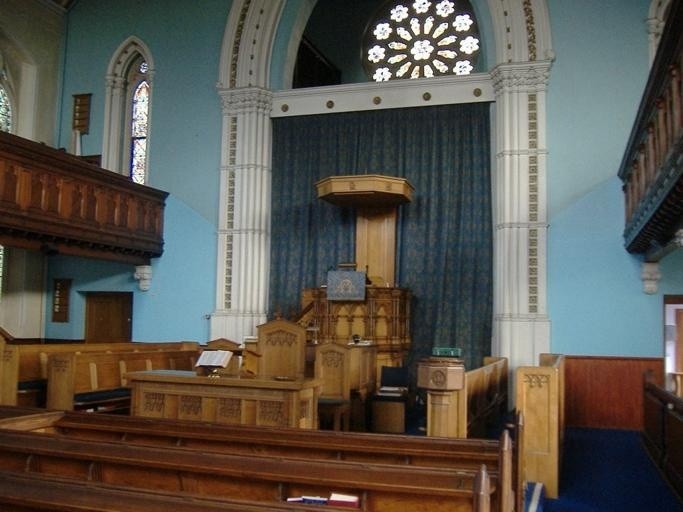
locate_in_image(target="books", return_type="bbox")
[432,347,465,357]
[327,492,360,508]
[377,386,409,397]
[302,496,329,505]
[286,497,303,504]
[195,350,234,368]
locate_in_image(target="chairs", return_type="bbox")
[312,342,351,432]
[206,337,241,375]
[256,319,306,379]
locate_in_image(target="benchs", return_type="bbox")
[1,340,200,407]
[0,471,343,512]
[45,351,200,412]
[0,429,491,512]
[53,415,514,512]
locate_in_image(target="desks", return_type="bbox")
[122,369,322,429]
